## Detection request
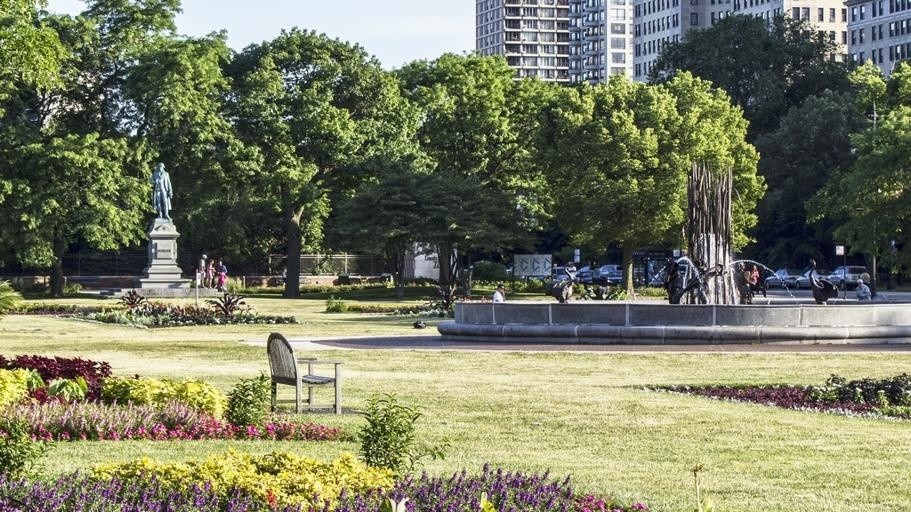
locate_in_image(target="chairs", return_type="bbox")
[267,331,345,415]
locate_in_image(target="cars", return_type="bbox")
[763,265,870,290]
[553,264,665,287]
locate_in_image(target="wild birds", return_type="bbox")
[547,264,582,301]
[802,257,840,303]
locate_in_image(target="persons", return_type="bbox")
[197,254,208,290]
[748,264,767,298]
[205,258,216,288]
[855,279,872,302]
[148,161,174,220]
[214,260,229,292]
[492,283,506,303]
